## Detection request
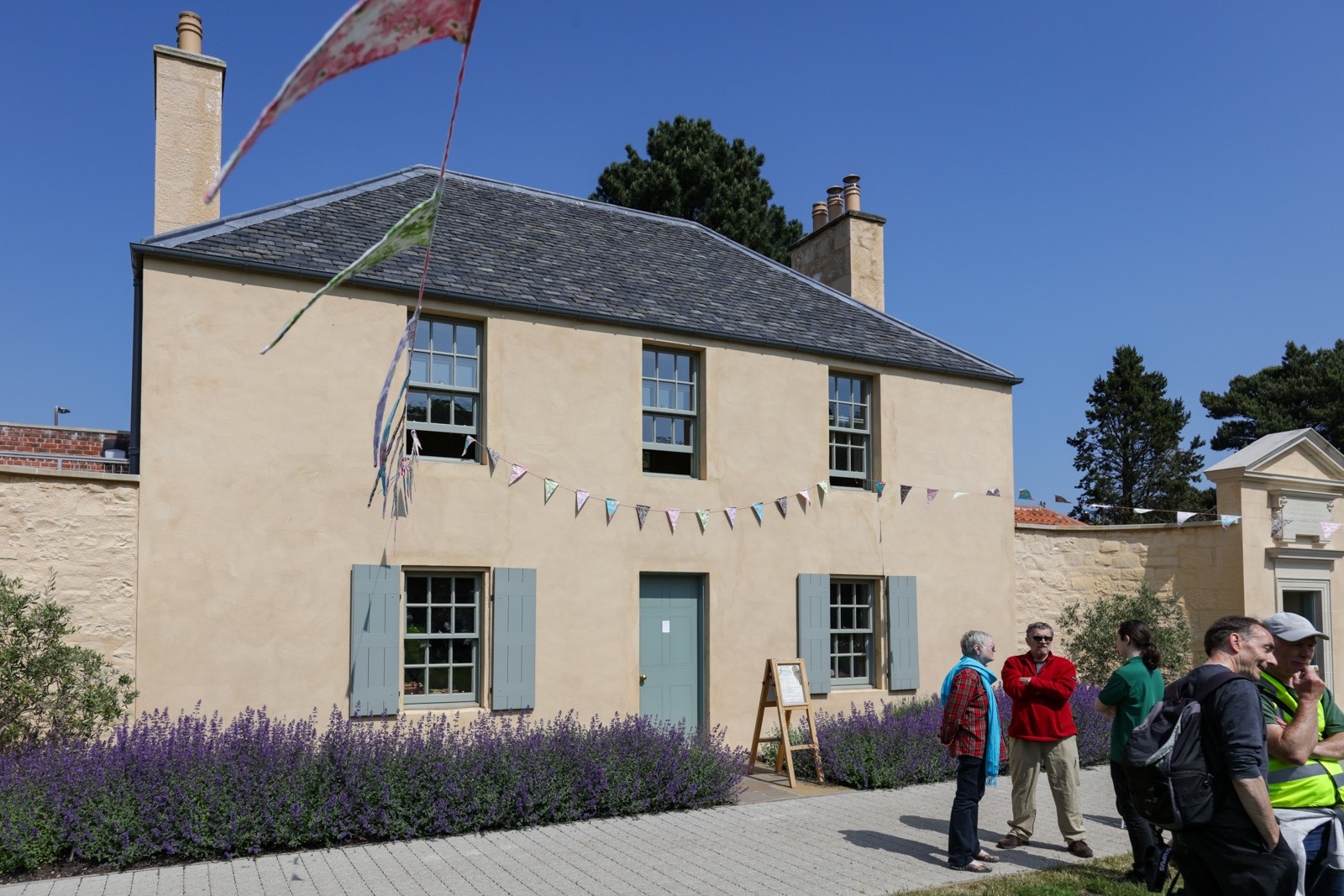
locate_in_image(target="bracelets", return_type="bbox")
[1028,677,1031,682]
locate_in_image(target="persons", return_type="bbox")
[936,630,1000,873]
[1256,612,1344,896]
[1162,616,1300,896]
[1093,619,1165,881]
[998,622,1093,858]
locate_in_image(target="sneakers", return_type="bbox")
[1068,840,1093,858]
[998,834,1029,848]
[1112,869,1171,885]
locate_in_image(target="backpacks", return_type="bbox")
[1122,672,1251,831]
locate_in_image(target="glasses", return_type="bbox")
[1028,636,1052,641]
[988,644,994,652]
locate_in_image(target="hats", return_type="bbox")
[1261,611,1330,642]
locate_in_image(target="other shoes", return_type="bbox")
[950,860,993,873]
[974,849,1000,862]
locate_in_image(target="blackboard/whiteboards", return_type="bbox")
[762,658,812,707]
